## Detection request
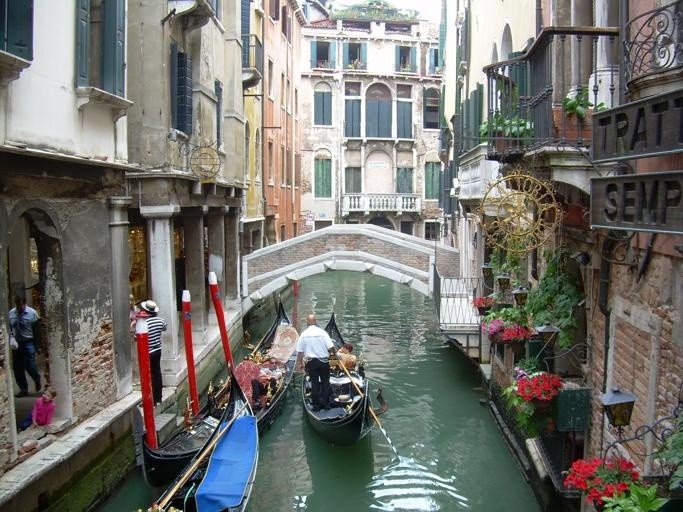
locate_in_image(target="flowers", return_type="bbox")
[471,296,640,512]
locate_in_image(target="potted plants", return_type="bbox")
[480,91,607,156]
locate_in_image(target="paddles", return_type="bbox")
[335,353,401,460]
[158,400,249,509]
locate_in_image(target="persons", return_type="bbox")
[295,313,338,412]
[334,344,356,376]
[6,294,41,399]
[15,384,56,434]
[128,294,139,341]
[136,299,165,411]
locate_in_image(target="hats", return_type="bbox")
[332,394,353,404]
[140,300,159,313]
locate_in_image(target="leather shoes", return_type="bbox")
[253,400,272,407]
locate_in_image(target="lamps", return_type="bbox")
[481,262,671,456]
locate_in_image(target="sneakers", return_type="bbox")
[15,373,41,397]
